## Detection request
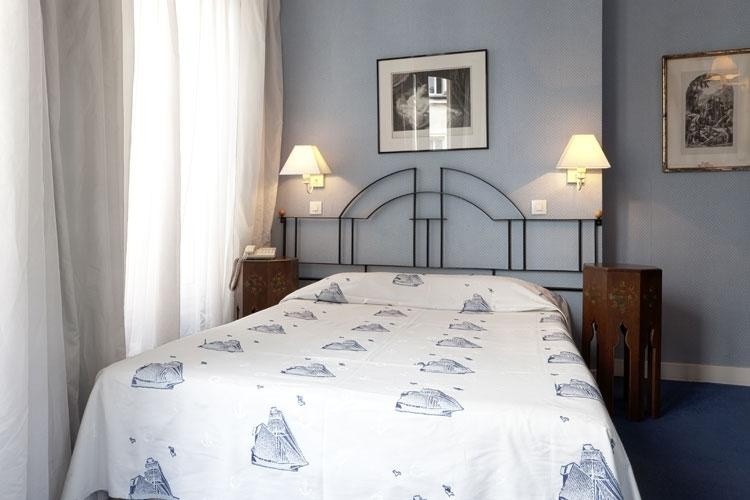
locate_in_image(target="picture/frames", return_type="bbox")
[376,48,489,154]
[662,48,750,174]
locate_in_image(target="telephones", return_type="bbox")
[242,245,278,259]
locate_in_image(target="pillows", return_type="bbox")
[287,272,562,313]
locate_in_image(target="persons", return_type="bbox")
[401,78,464,130]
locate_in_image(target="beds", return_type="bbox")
[56,166,642,500]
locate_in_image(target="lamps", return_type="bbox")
[556,134,612,191]
[279,144,332,195]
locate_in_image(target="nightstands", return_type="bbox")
[581,263,662,420]
[241,257,299,317]
